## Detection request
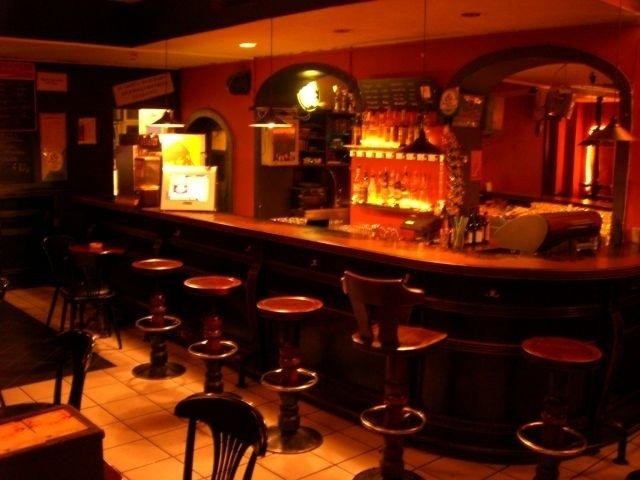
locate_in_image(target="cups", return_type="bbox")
[453,228,464,251]
[439,228,449,248]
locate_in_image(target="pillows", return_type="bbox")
[0,298,117,393]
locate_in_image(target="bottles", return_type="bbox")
[357,108,415,146]
[331,89,354,114]
[351,163,430,210]
[467,208,490,245]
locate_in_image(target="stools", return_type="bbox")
[257,297,325,454]
[132,257,184,379]
[184,273,243,393]
[517,338,601,479]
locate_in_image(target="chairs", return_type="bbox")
[0,331,94,420]
[175,394,267,478]
[42,235,123,351]
[341,272,446,480]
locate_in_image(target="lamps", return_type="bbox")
[151,40,186,128]
[590,0,636,143]
[394,1,447,154]
[250,18,291,128]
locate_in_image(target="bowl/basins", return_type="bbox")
[290,181,328,210]
